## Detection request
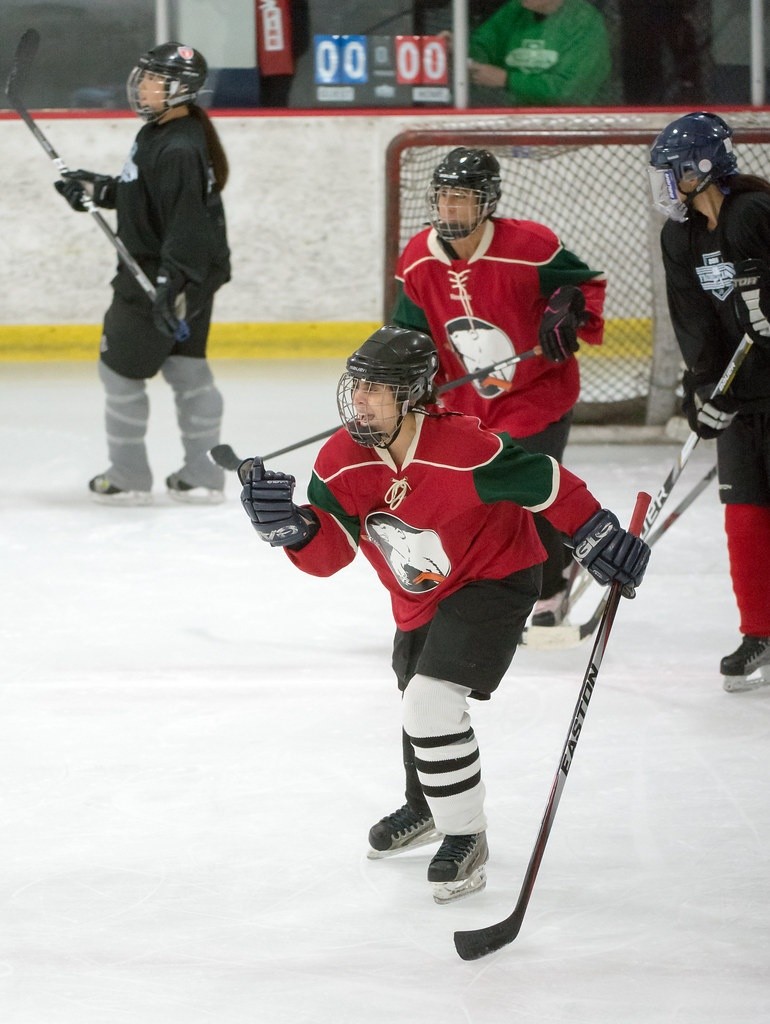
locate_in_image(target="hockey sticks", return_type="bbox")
[4,27,193,342]
[206,343,544,472]
[451,491,654,962]
[646,464,717,551]
[525,334,756,645]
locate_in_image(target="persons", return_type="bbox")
[646,111,770,694]
[468,0,612,108]
[243,329,650,903]
[54,41,225,504]
[387,146,608,629]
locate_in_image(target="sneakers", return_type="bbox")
[165,472,223,506]
[88,473,152,505]
[532,587,565,627]
[366,801,444,861]
[720,635,770,692]
[428,827,492,904]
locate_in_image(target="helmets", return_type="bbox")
[125,41,209,118]
[336,325,441,449]
[424,147,502,242]
[648,112,736,223]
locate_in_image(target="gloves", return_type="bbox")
[240,456,313,547]
[560,508,651,600]
[538,285,584,363]
[152,264,186,335]
[54,167,116,212]
[730,258,769,347]
[680,371,740,441]
